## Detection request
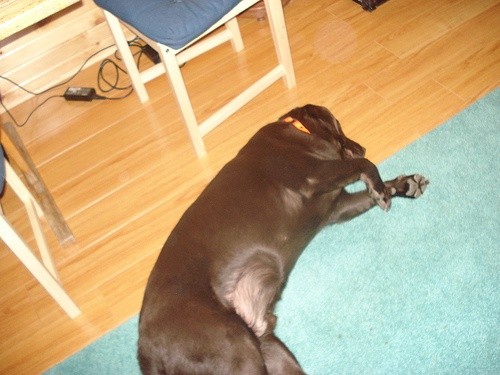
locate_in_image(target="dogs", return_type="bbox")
[135,102,429,375]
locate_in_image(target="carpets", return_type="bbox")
[39,83,500,375]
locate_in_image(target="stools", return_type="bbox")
[0,0,296,320]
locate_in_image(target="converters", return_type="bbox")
[64,87,96,101]
[142,44,161,64]
